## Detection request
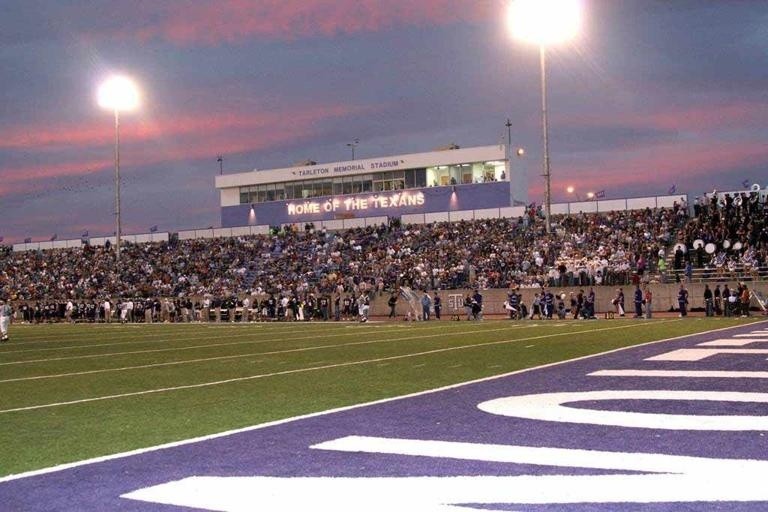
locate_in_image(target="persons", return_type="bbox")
[2,170,768,326]
[0,301,12,344]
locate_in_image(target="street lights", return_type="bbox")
[92,69,146,259]
[502,0,583,234]
